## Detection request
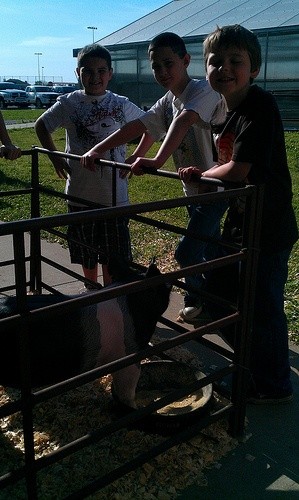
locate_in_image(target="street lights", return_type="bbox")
[88,26,97,43]
[35,52,42,81]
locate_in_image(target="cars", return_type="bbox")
[8,79,75,97]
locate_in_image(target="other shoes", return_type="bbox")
[178,305,200,320]
[246,389,293,405]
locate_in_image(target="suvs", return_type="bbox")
[25,85,61,109]
[0,82,30,108]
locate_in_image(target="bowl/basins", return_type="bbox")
[111,360,213,417]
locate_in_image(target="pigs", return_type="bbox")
[0,263,172,401]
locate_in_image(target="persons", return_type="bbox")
[177,23,299,405]
[0,112,21,160]
[80,32,224,322]
[35,45,157,290]
